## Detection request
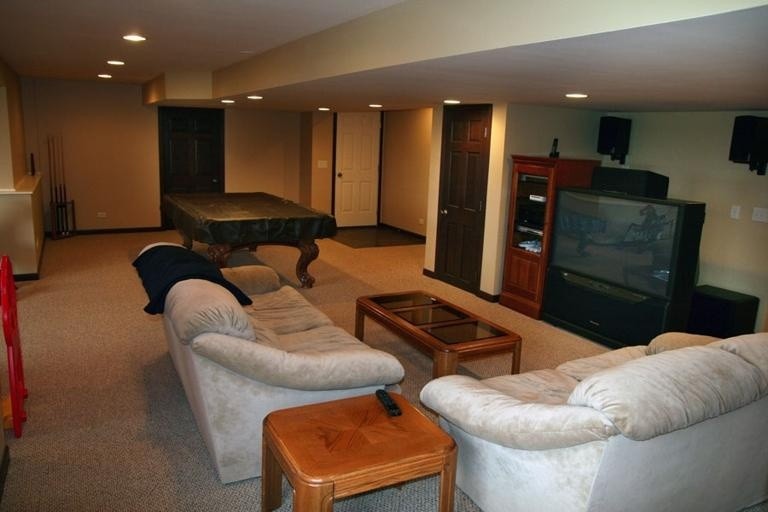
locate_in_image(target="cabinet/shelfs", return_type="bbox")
[501,155,602,321]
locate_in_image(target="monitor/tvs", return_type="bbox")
[547,185,706,299]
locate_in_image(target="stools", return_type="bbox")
[261,391,458,510]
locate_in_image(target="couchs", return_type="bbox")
[419,332,768,512]
[133,242,404,485]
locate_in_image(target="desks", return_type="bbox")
[164,191,336,289]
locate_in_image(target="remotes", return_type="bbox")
[376,388,402,418]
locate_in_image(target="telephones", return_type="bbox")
[550,138,560,157]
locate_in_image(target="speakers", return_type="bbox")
[728,114,768,176]
[597,116,632,164]
